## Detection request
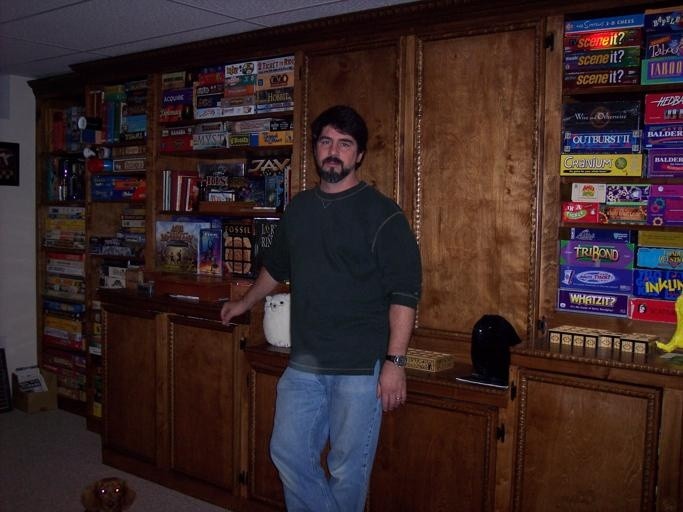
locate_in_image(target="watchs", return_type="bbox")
[384,351,409,370]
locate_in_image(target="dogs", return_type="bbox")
[80,477,137,512]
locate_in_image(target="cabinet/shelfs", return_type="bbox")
[103,293,243,494]
[508,355,682,512]
[153,47,304,276]
[87,56,159,425]
[543,0,683,359]
[34,74,88,409]
[249,352,505,510]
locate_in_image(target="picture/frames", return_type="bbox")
[0,141,20,188]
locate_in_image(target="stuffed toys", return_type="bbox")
[259,290,294,349]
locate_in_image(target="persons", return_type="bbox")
[215,103,422,511]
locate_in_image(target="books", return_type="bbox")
[12,364,49,396]
[154,56,295,282]
[37,79,152,414]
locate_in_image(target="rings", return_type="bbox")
[394,395,402,401]
